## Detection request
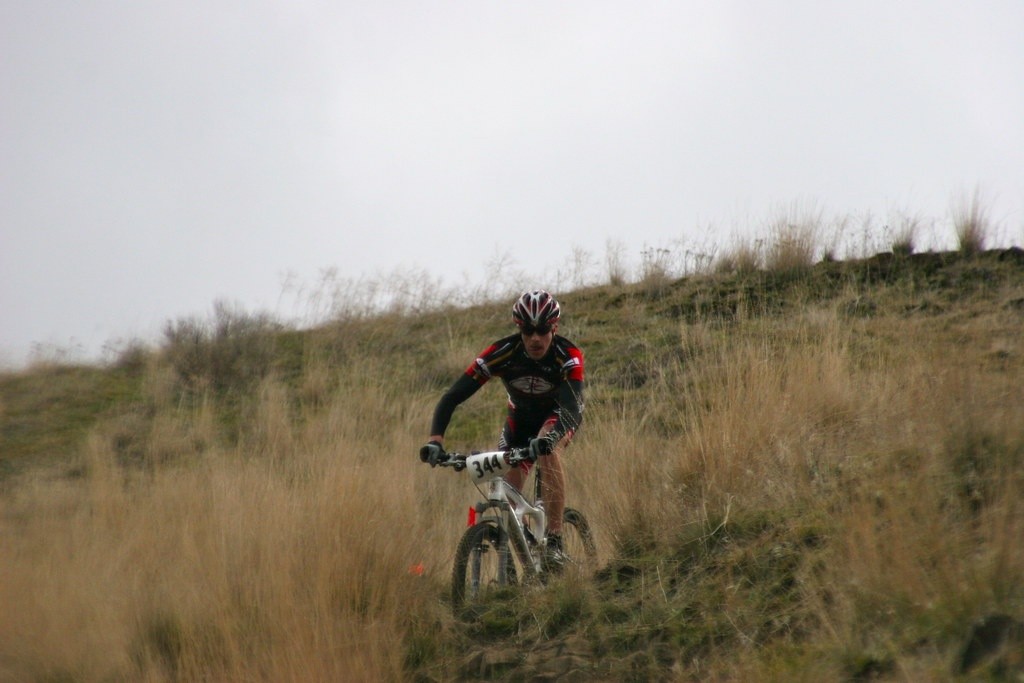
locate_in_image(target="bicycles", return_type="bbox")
[435,437,598,634]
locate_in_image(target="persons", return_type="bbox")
[420,291,585,574]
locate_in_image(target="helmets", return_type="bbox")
[511,290,561,324]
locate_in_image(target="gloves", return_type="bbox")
[528,436,555,461]
[419,440,450,468]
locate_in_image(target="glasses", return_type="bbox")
[521,322,554,336]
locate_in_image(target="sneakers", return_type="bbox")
[539,549,565,574]
